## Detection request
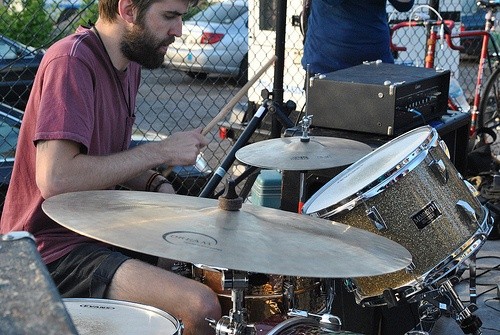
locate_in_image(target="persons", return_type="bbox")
[0,0,222,335]
[301,0,416,75]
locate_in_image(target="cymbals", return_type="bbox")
[235,136,372,170]
[41,190,412,278]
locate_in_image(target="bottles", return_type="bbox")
[436,66,471,113]
[196,152,204,167]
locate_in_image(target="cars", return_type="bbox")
[0,34,48,113]
[461,8,496,61]
[162,0,248,87]
[0,102,25,222]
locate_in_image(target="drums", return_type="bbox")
[0,230,79,335]
[300,125,495,308]
[234,309,363,335]
[191,261,335,324]
[61,297,185,335]
